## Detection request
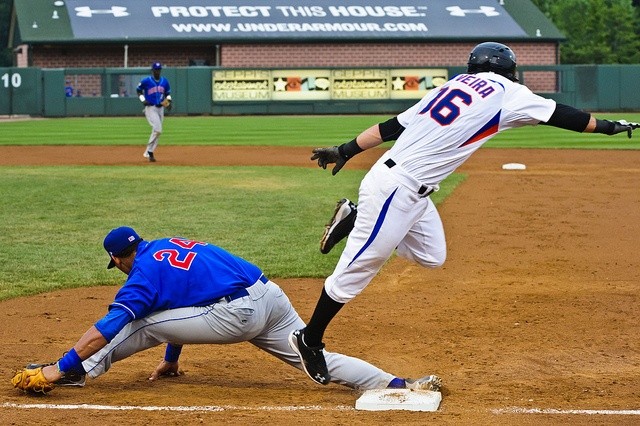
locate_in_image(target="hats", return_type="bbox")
[152,63,162,68]
[102,226,141,268]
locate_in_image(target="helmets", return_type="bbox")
[467,41,519,82]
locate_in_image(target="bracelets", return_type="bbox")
[166,95,171,100]
[165,343,183,362]
[139,95,145,102]
[58,348,84,373]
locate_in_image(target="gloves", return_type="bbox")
[142,100,150,105]
[311,145,347,175]
[609,120,640,139]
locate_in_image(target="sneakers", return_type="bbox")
[25,363,87,388]
[148,151,156,162]
[287,327,330,385]
[402,374,442,396]
[320,198,357,254]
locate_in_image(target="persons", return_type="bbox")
[288,41,639,387]
[10,226,443,397]
[76,90,82,97]
[65,80,73,97]
[136,62,172,162]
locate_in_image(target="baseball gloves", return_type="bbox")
[11,366,58,395]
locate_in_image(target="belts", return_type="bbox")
[384,159,433,198]
[226,273,269,302]
